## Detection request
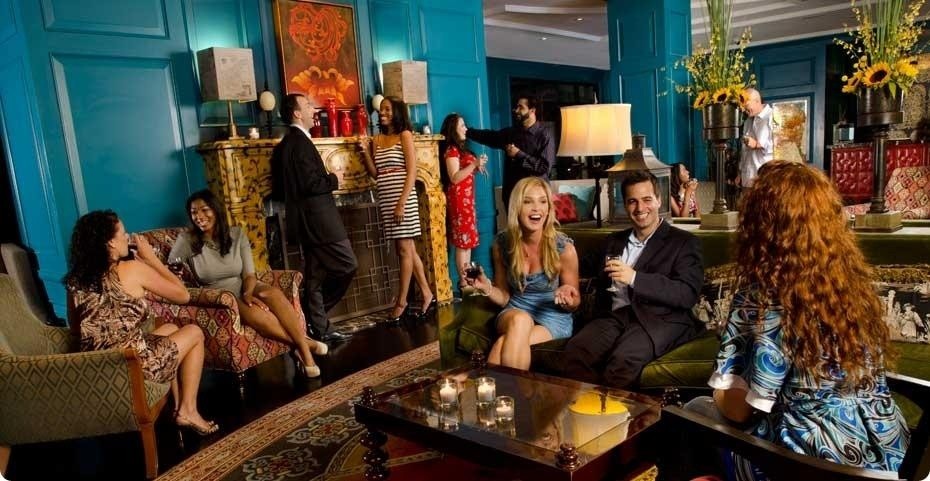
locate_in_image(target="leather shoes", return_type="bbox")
[302,304,320,340]
[320,331,353,344]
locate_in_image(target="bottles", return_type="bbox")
[310,99,368,138]
[849,215,856,229]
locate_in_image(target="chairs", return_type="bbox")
[660,368,930,481]
[0,272,172,479]
[131,224,308,387]
[0,242,67,328]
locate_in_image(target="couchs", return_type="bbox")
[543,176,719,221]
[438,229,930,398]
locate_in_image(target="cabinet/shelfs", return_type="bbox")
[826,140,930,203]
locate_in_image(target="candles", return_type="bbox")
[497,400,511,419]
[249,128,259,140]
[477,384,494,401]
[445,411,458,428]
[440,378,456,403]
[478,407,496,427]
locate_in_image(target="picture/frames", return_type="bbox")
[761,92,815,164]
[273,0,367,125]
[759,56,816,92]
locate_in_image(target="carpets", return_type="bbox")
[152,340,658,481]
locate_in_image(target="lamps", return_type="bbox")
[258,90,277,138]
[382,60,428,135]
[563,390,631,458]
[372,93,386,134]
[605,132,674,226]
[196,46,258,140]
[555,102,633,227]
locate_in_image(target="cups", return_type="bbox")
[141,311,156,333]
[437,378,458,410]
[480,155,486,163]
[248,127,259,141]
[475,377,496,410]
[715,318,727,343]
[496,397,515,423]
[423,126,431,135]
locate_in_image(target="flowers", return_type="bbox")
[832,1,925,96]
[657,0,758,113]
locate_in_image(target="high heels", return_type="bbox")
[304,336,328,355]
[384,302,409,327]
[415,293,437,322]
[172,410,214,426]
[293,348,321,378]
[175,416,220,441]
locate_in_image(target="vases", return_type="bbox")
[354,104,369,136]
[327,97,339,137]
[310,108,324,138]
[339,110,354,136]
[696,102,742,230]
[856,82,908,233]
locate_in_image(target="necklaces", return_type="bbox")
[523,243,539,257]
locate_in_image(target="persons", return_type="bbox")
[560,170,706,390]
[166,189,328,378]
[682,159,912,481]
[515,372,584,461]
[465,95,554,217]
[439,114,489,296]
[670,163,700,218]
[463,175,581,370]
[270,94,359,341]
[61,211,220,441]
[357,96,437,325]
[734,88,781,211]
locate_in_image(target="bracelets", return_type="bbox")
[481,279,492,297]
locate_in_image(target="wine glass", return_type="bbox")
[168,256,184,282]
[463,262,482,297]
[604,256,622,293]
[127,233,160,258]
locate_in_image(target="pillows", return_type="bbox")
[696,261,746,337]
[558,184,596,221]
[592,183,618,222]
[862,265,930,346]
[553,193,577,223]
